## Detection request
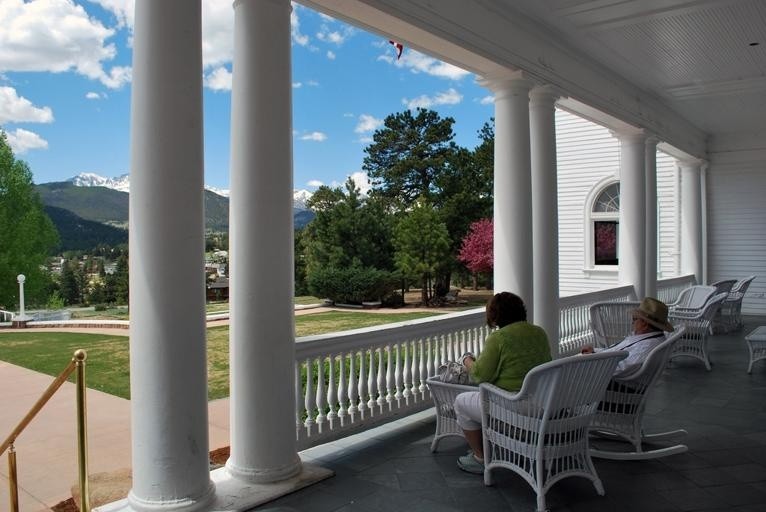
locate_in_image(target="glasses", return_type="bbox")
[632,317,639,321]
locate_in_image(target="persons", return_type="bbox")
[579,296,676,413]
[453,290,551,475]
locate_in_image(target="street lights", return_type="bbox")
[16,273,27,317]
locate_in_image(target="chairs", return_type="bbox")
[744,324,766,374]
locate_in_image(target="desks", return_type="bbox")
[426,361,483,455]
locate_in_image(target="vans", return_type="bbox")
[0,311,14,327]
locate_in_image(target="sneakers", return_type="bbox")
[456,453,483,475]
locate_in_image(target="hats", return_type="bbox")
[625,297,674,332]
